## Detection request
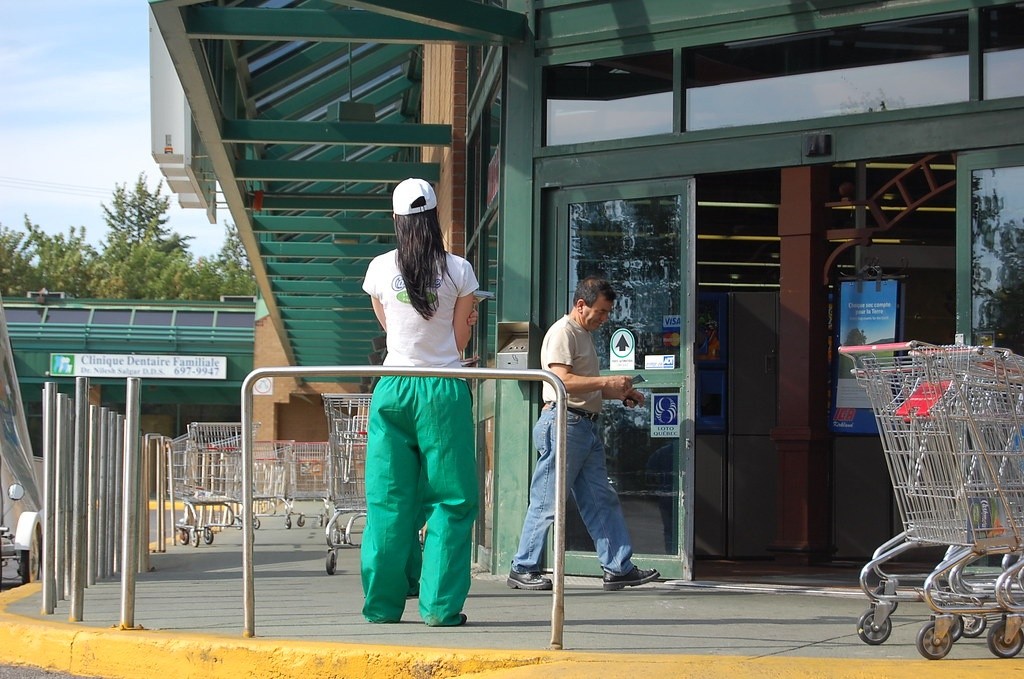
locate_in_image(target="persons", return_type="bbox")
[359,176,479,627]
[507,276,661,590]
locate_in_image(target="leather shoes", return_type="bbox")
[603,565,660,591]
[506,568,553,590]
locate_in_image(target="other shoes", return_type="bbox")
[460,614,467,625]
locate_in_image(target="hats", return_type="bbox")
[393,178,437,215]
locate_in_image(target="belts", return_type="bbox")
[546,401,599,422]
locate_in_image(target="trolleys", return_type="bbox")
[834,338,1024,657]
[168,391,426,576]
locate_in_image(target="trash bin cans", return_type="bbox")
[2,456,43,543]
